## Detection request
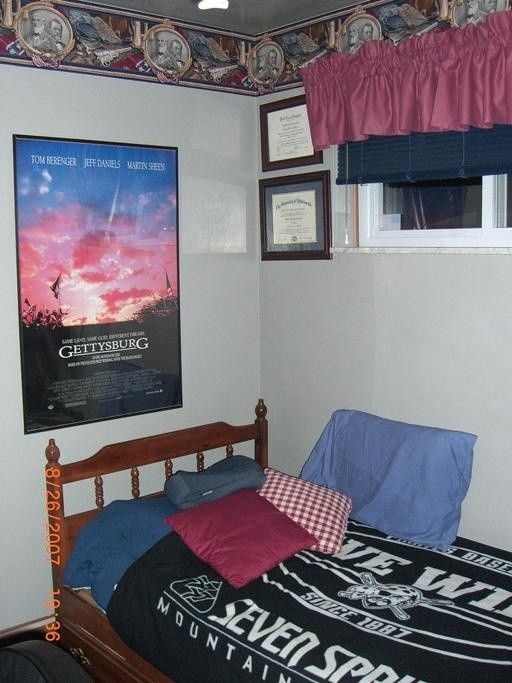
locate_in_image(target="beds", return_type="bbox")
[45,399,512,683]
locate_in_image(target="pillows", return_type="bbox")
[163,455,262,510]
[297,409,478,549]
[166,487,315,588]
[258,463,353,554]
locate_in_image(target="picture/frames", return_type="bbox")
[259,169,333,260]
[13,134,183,435]
[259,93,323,172]
